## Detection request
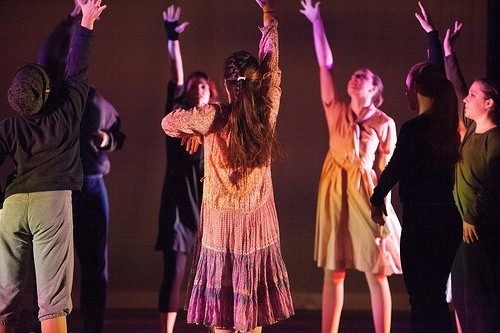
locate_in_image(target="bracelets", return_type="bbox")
[263,10,275,14]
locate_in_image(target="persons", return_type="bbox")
[39,0,128,333]
[161,1,295,333]
[0,0,109,333]
[368,2,465,332]
[443,20,500,333]
[297,2,405,333]
[156,6,219,331]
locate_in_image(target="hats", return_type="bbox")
[408,61,456,99]
[7,64,53,116]
